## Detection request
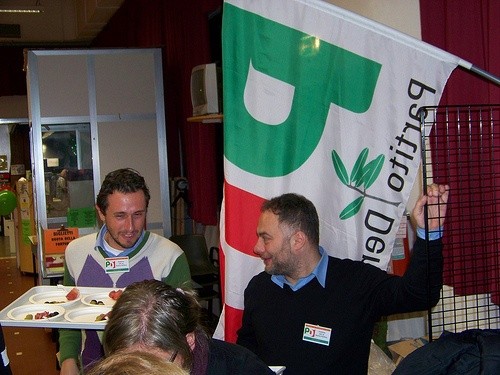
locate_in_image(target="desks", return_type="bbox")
[28,236,38,286]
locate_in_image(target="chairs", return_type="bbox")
[170,234,223,334]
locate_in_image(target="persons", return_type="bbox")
[237,183,449,375]
[103,279,278,375]
[59,169,191,374]
[56,169,67,198]
[86,351,191,375]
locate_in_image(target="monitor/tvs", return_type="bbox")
[190,63,222,116]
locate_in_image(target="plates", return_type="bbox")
[64,306,112,323]
[81,293,118,307]
[29,291,82,306]
[7,304,65,321]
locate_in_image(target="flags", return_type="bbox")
[212,0,458,343]
